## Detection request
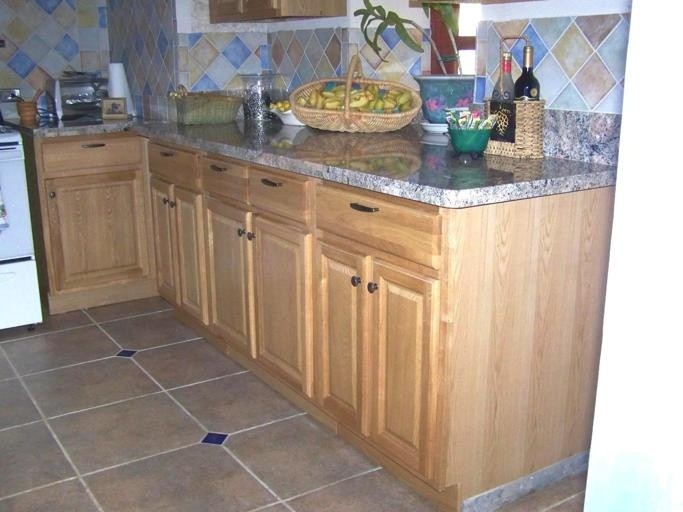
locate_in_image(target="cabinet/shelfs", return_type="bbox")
[146,140,211,329]
[310,184,441,485]
[41,136,148,295]
[198,152,314,400]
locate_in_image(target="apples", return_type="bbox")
[272,138,294,150]
[273,100,290,111]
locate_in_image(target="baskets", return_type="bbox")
[483,34,545,160]
[288,54,423,133]
[167,84,244,126]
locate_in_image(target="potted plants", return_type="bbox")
[352,0,475,125]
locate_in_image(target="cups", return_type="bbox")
[16,102,37,120]
[20,119,37,130]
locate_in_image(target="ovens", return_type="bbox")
[0,126,44,332]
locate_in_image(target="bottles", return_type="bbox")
[240,72,283,123]
[244,123,281,151]
[491,44,539,104]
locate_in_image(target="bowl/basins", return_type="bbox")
[447,155,490,186]
[268,107,307,126]
[447,128,491,153]
[271,126,305,146]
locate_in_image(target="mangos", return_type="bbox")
[297,84,413,112]
[354,111,355,113]
